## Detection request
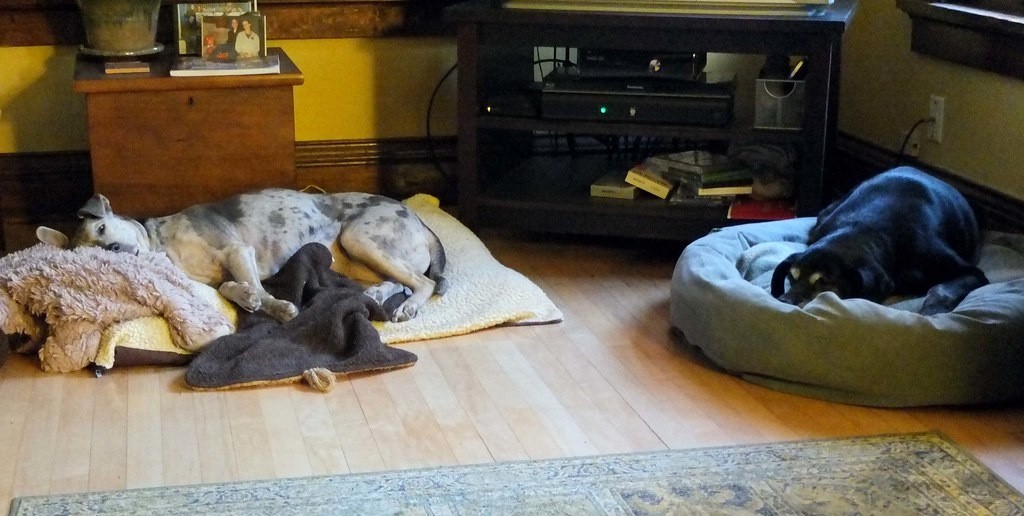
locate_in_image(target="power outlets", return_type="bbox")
[926,95,944,144]
[901,131,920,157]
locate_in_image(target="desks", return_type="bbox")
[72,47,304,217]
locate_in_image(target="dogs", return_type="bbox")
[771,166,989,318]
[36,186,446,323]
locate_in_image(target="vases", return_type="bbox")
[76,0,165,57]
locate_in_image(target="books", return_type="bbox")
[170,56,280,74]
[648,150,754,204]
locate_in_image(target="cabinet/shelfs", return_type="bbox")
[457,0,858,235]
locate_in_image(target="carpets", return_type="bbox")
[8,429,1024,516]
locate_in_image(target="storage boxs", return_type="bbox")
[592,162,677,200]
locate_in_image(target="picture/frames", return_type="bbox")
[177,0,267,63]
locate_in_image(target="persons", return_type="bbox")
[183,3,258,58]
